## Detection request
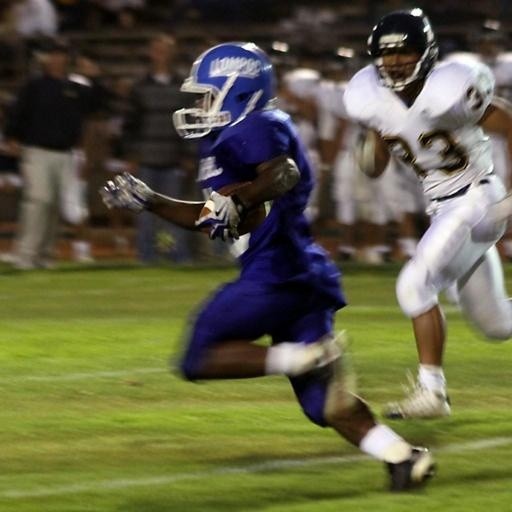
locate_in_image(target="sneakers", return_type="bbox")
[383,386,451,418]
[386,447,438,492]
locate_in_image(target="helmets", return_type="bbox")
[368,13,439,93]
[173,42,278,142]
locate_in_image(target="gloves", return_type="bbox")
[100,171,152,212]
[196,191,243,244]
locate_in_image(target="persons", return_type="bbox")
[342,8,512,421]
[0,1,511,269]
[97,43,437,490]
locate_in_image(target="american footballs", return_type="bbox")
[195,182,274,237]
